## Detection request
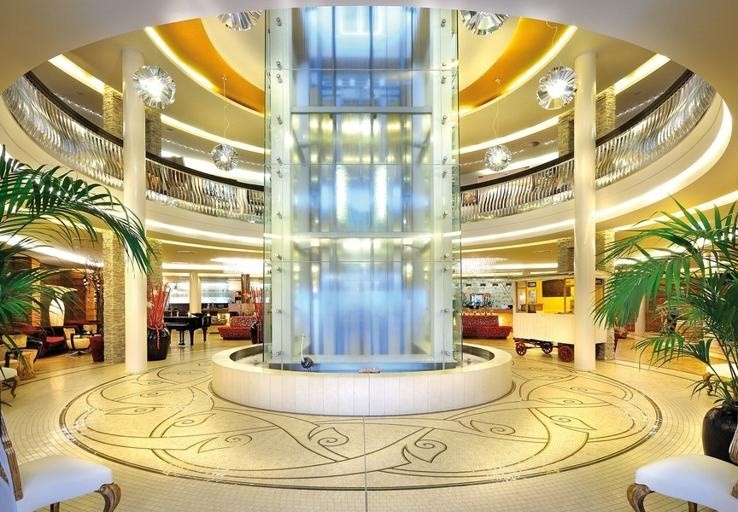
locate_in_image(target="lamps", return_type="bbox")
[479,77,512,172]
[535,18,577,112]
[210,73,239,170]
[131,60,176,112]
[457,7,508,34]
[215,10,263,34]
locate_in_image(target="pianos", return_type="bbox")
[164,313,211,346]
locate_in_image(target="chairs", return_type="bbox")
[1,333,42,370]
[633,424,738,512]
[0,409,122,512]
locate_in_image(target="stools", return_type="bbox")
[699,362,738,395]
[1,366,20,400]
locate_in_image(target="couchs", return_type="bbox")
[26,323,100,359]
[460,312,509,339]
[218,314,256,339]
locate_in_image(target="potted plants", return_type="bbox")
[595,196,737,467]
